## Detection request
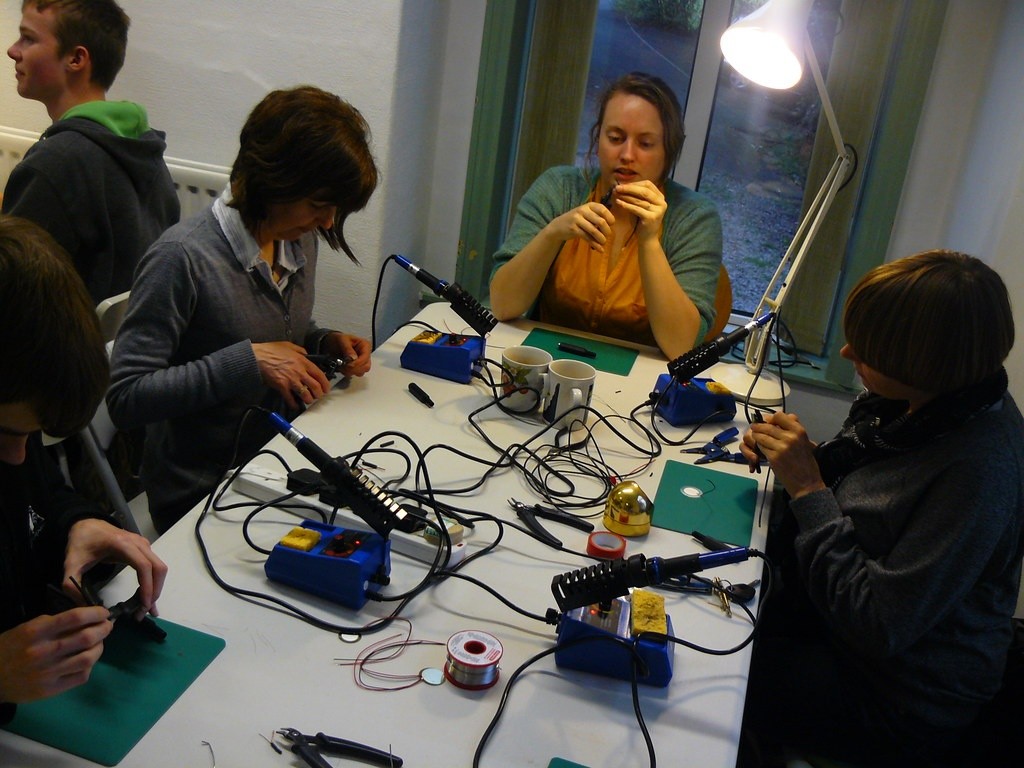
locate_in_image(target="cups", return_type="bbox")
[542,359,598,431]
[499,345,553,412]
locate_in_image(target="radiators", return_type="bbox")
[0,126,232,223]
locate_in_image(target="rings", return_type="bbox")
[645,202,651,211]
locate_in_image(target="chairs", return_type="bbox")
[42,290,160,545]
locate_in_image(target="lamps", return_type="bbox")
[710,1,852,406]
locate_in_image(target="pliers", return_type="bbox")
[507,497,594,547]
[299,353,344,395]
[275,727,403,768]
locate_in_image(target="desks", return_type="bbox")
[0,302,785,767]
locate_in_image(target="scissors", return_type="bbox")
[679,426,768,466]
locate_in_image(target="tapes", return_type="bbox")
[586,531,627,560]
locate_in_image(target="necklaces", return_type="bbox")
[271,239,280,275]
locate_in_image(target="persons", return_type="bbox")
[0,0,180,311]
[0,215,167,728]
[102,87,377,535]
[739,248,1024,768]
[488,69,722,359]
